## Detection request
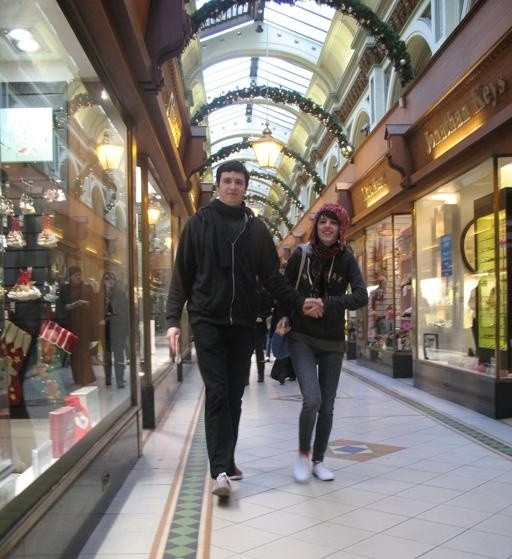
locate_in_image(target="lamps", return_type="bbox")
[148,197,161,225]
[95,128,127,171]
[249,0,285,169]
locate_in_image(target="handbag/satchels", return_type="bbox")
[272,332,290,360]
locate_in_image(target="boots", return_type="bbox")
[257,366,265,382]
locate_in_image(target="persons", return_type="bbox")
[251,287,273,383]
[61,266,96,384]
[369,277,392,350]
[267,316,296,384]
[274,203,368,484]
[96,271,128,388]
[165,161,325,497]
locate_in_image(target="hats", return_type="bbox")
[309,204,349,250]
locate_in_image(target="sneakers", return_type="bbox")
[230,468,242,480]
[211,473,232,497]
[117,382,124,387]
[311,462,335,481]
[107,377,111,384]
[294,454,309,482]
